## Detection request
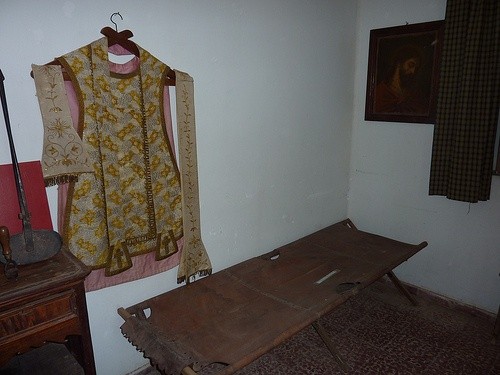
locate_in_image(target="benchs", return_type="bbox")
[117,218,429,375]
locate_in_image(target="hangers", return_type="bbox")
[29,12,176,86]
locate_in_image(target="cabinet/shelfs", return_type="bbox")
[0,248,97,375]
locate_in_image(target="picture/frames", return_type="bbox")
[364,19,446,123]
